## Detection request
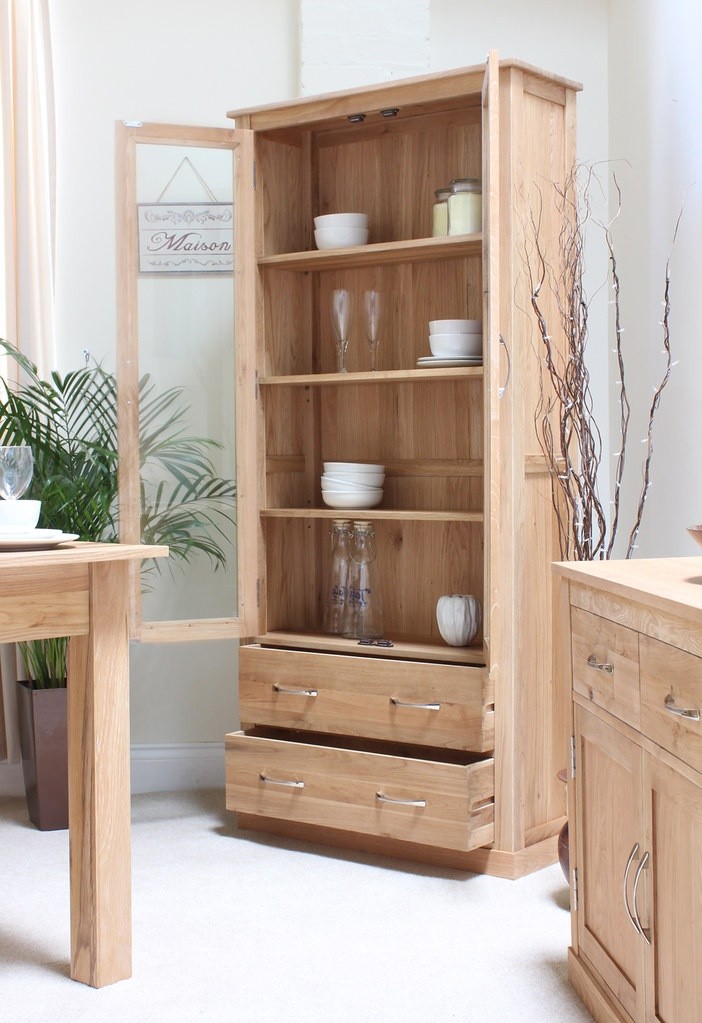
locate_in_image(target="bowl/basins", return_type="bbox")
[313,212,370,251]
[428,320,483,356]
[0,499,42,541]
[320,461,386,510]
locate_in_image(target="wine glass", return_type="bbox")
[0,442,35,501]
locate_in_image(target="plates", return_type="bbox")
[0,533,80,552]
[416,356,483,368]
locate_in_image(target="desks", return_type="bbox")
[0,540,170,990]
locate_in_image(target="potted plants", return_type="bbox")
[0,337,237,826]
[503,158,692,886]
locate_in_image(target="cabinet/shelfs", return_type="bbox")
[109,47,589,879]
[559,555,702,1023]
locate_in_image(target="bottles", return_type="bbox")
[343,520,384,638]
[447,177,483,238]
[322,519,358,635]
[432,188,453,237]
